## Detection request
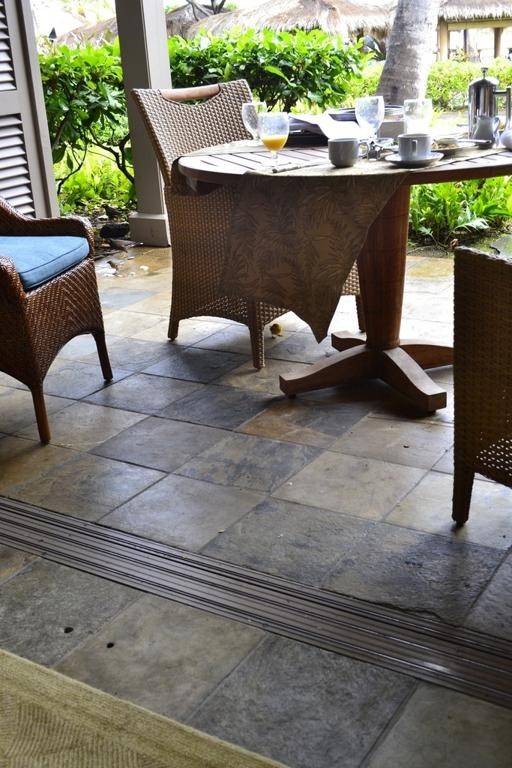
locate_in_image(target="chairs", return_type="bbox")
[128,78,367,370]
[450,241,511,527]
[0,193,113,444]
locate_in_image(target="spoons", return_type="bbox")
[439,138,492,144]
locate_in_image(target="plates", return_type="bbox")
[384,141,476,165]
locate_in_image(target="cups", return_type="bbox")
[354,96,433,141]
[327,133,433,167]
[468,67,510,140]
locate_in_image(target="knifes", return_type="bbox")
[271,162,326,173]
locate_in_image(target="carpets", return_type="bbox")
[0,647,290,767]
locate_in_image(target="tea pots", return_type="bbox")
[472,115,500,149]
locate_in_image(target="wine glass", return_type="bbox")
[241,101,290,166]
